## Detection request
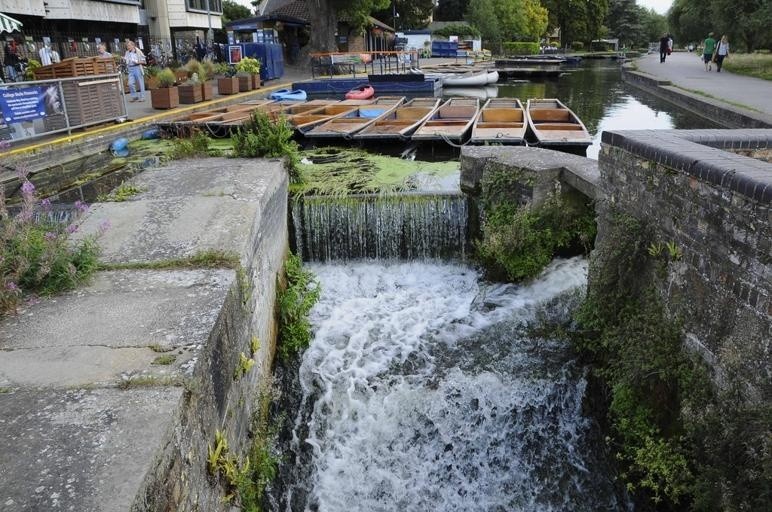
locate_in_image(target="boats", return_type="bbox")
[280,89,307,101]
[353,98,445,151]
[344,85,376,101]
[156,99,342,141]
[268,88,290,99]
[471,97,527,145]
[409,67,500,86]
[527,98,594,151]
[305,96,408,148]
[411,96,480,152]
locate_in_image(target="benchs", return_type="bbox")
[311,54,374,79]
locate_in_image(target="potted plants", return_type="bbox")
[144,54,261,108]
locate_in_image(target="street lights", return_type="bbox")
[195,30,201,63]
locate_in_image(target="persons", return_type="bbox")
[658,32,666,61]
[702,32,716,73]
[716,34,730,71]
[666,37,673,55]
[123,42,148,102]
[49,47,61,64]
[98,44,112,58]
[39,45,53,67]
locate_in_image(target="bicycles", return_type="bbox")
[115,46,195,75]
[0,63,33,83]
